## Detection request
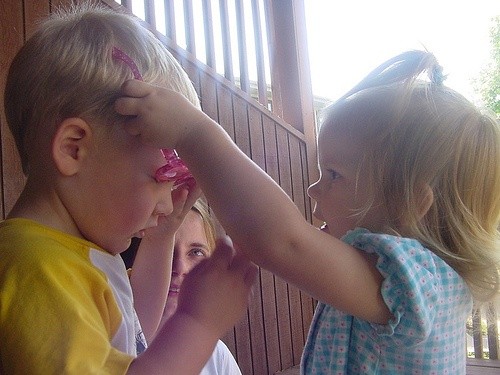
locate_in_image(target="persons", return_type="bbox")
[114,43,497,374]
[138,189,249,375]
[0,5,261,375]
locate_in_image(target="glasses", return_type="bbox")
[109,47,196,190]
